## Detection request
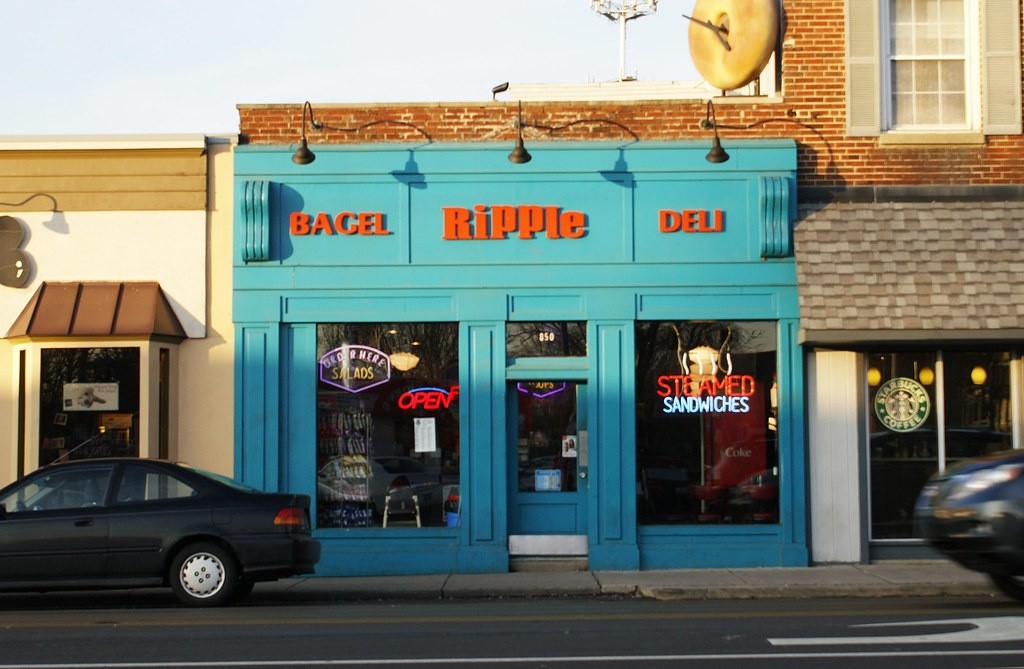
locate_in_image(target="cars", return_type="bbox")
[519,455,568,492]
[703,438,779,522]
[0,457,321,608]
[915,449,1024,603]
[869,428,1012,502]
[317,456,442,514]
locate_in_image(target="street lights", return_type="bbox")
[688,337,719,512]
[377,328,398,351]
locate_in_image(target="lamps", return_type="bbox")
[702,100,728,163]
[291,102,321,166]
[507,99,532,165]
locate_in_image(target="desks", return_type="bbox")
[383,488,420,527]
